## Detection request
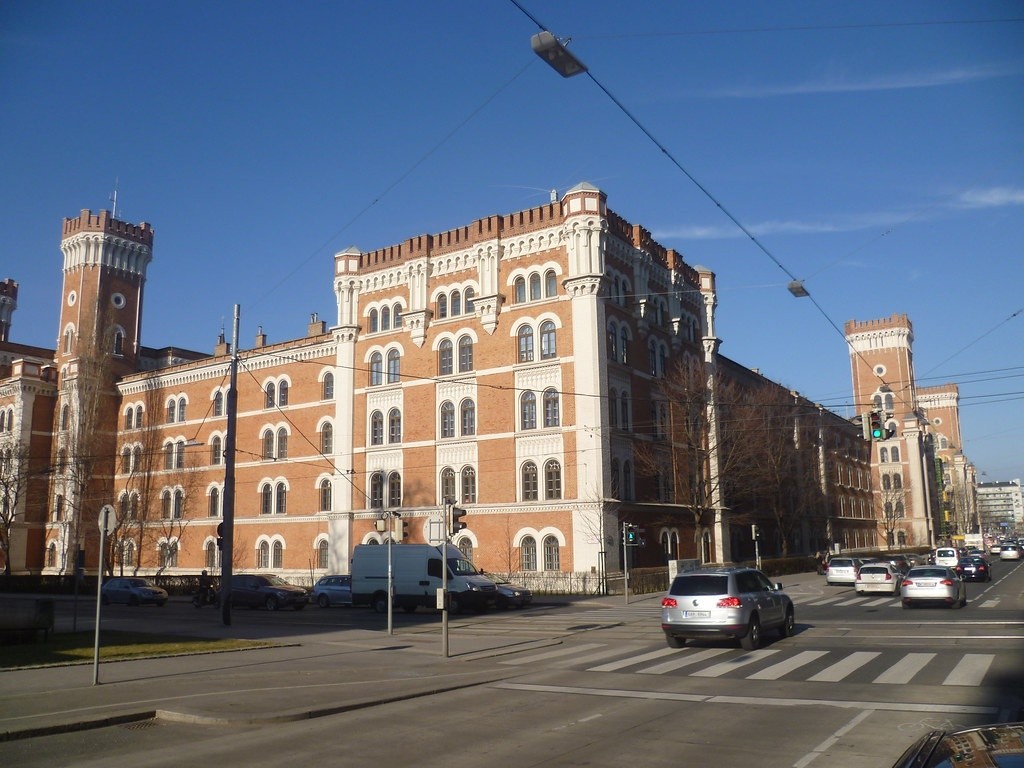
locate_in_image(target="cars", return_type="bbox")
[825,541,1023,605]
[100,577,170,607]
[478,571,532,610]
[310,576,352,609]
[894,725,1022,768]
[660,570,795,644]
[231,573,309,612]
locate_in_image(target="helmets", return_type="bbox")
[202,570,207,575]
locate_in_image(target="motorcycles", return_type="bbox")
[191,582,218,609]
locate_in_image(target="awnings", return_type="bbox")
[604,498,731,511]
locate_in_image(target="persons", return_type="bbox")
[815,551,830,575]
[447,560,458,571]
[196,570,217,609]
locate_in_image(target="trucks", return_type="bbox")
[349,544,495,616]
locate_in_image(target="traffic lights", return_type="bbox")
[855,412,870,442]
[449,504,467,536]
[885,411,896,440]
[395,517,408,541]
[868,410,883,440]
